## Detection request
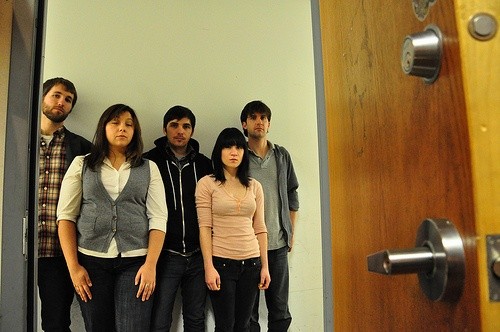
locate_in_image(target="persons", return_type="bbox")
[195,127,271,332]
[38,77,94,332]
[57,103,168,332]
[142,106,214,332]
[240,101,300,332]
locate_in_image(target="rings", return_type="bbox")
[146,285,149,287]
